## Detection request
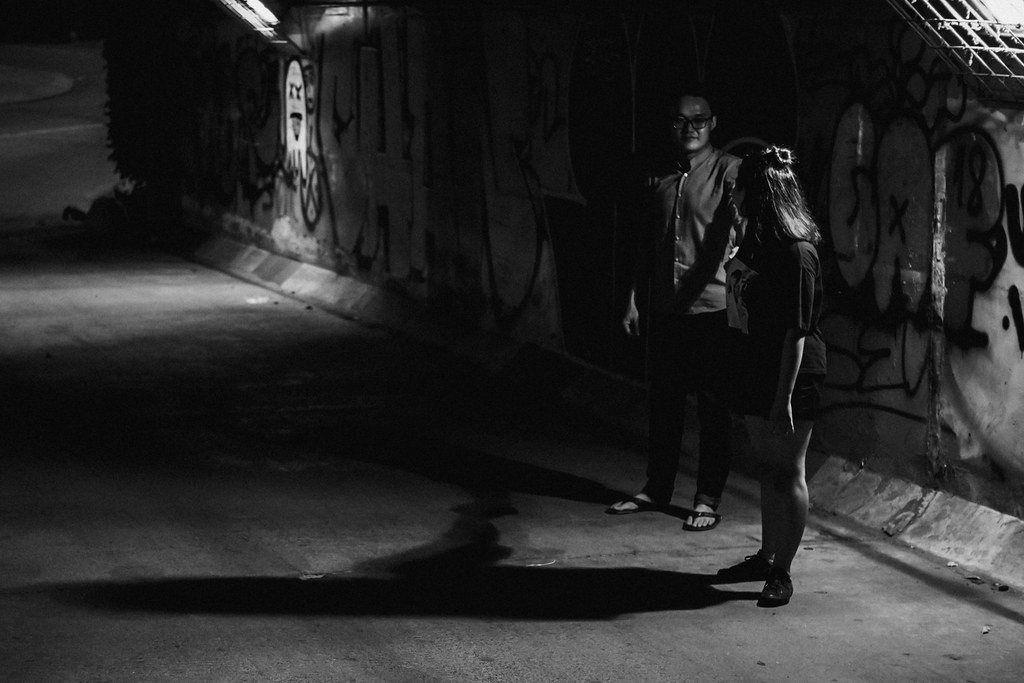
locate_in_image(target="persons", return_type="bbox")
[717,147,828,606]
[608,79,744,530]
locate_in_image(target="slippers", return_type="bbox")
[608,497,674,514]
[683,509,724,532]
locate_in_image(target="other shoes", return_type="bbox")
[760,568,794,608]
[717,554,774,583]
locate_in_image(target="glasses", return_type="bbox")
[672,114,714,129]
